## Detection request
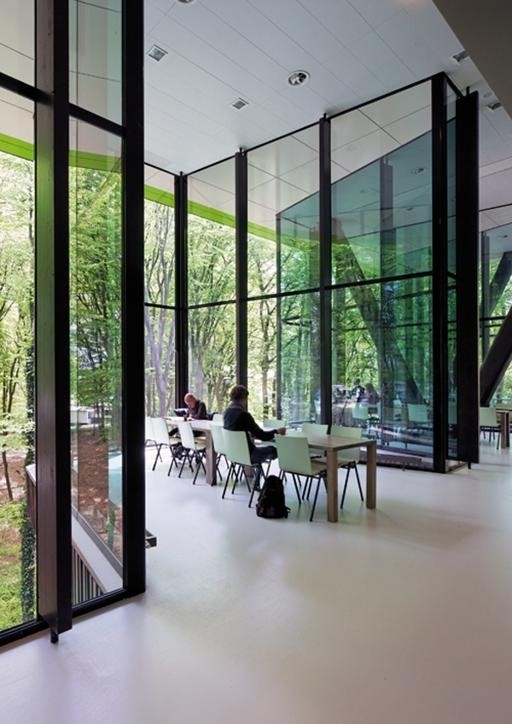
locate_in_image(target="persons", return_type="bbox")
[351,377,364,402]
[362,383,378,404]
[179,395,206,453]
[224,385,286,492]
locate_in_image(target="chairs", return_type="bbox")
[270,435,328,522]
[145,418,179,475]
[291,423,329,500]
[221,428,264,508]
[481,408,501,450]
[212,414,230,481]
[330,426,363,509]
[263,420,286,484]
[178,422,207,485]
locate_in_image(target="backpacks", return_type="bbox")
[258,476,291,519]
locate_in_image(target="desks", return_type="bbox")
[486,410,509,449]
[275,433,377,522]
[223,429,282,443]
[164,417,219,485]
